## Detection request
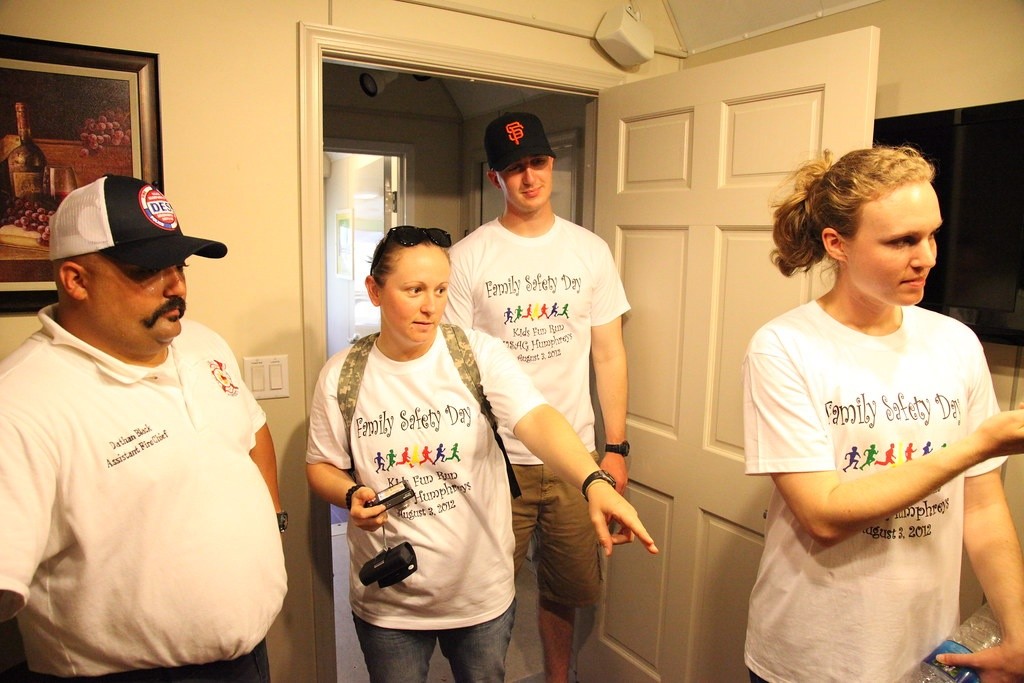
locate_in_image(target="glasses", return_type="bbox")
[371,226,452,276]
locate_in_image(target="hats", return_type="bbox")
[49,174,228,270]
[485,111,556,172]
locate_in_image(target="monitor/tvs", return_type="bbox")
[874,97,1024,347]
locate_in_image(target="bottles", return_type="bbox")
[7,103,48,199]
[907,601,1011,683]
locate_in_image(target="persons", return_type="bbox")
[0,176,286,682]
[744,148,1024,683]
[308,226,657,683]
[438,113,630,682]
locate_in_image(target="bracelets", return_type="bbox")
[346,485,367,511]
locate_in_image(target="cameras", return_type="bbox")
[365,480,416,517]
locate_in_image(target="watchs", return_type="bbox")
[582,469,617,502]
[276,512,288,533]
[605,440,631,457]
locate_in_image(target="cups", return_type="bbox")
[43,164,78,209]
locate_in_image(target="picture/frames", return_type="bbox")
[0,33,165,315]
[334,207,354,282]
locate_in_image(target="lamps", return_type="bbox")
[359,69,399,98]
